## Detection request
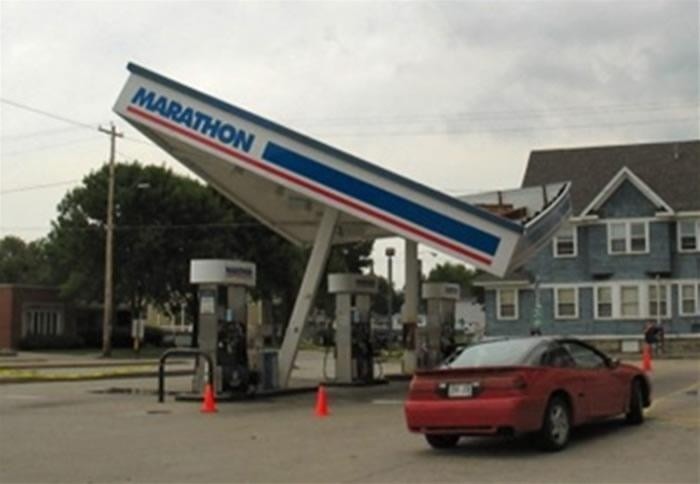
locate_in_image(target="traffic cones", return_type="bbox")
[312,386,330,417]
[642,346,653,372]
[199,383,217,413]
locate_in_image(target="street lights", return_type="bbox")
[385,247,395,354]
[419,250,438,259]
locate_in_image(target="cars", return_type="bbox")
[407,337,650,452]
